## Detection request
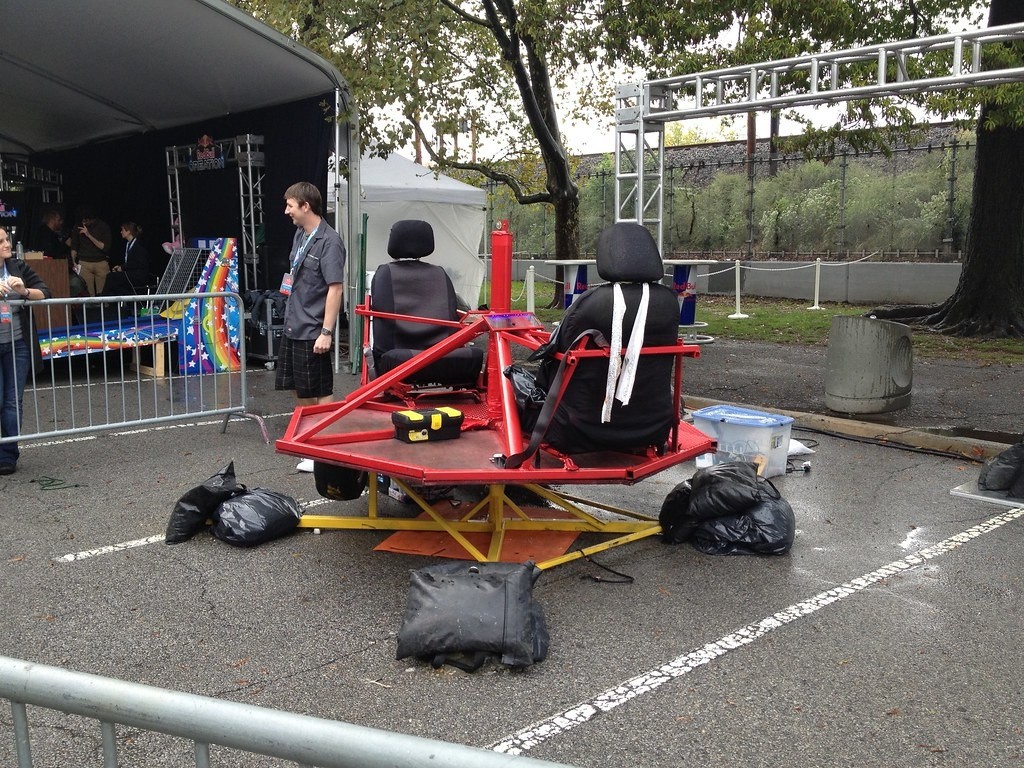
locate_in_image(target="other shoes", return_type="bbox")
[0,462,17,475]
[93,303,110,309]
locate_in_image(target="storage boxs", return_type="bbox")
[691,403,794,479]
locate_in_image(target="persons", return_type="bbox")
[34,206,149,308]
[0,226,52,475]
[283,183,347,473]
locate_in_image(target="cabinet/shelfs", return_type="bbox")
[242,294,284,372]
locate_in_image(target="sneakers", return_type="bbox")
[296,458,314,472]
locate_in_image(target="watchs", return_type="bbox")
[321,328,332,335]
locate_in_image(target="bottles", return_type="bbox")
[16,241,23,259]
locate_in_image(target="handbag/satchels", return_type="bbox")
[685,461,781,525]
[396,560,551,673]
[165,459,251,544]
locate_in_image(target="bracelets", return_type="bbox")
[21,288,31,298]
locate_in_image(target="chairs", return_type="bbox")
[369,221,484,396]
[513,226,679,465]
